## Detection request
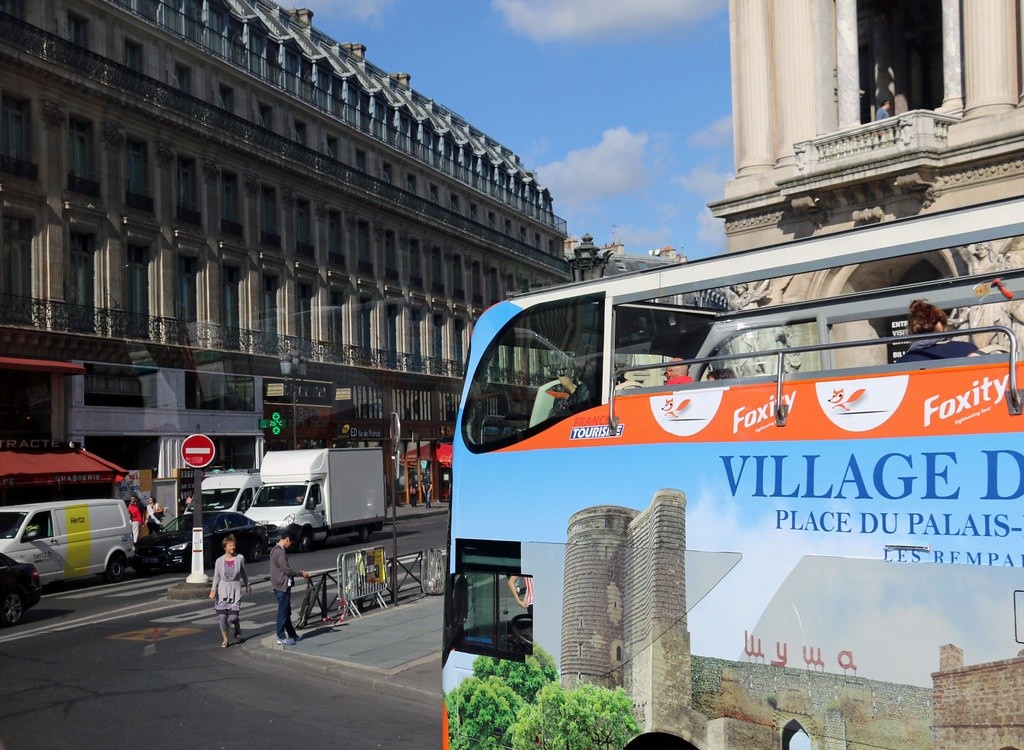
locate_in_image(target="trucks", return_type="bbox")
[243,447,389,552]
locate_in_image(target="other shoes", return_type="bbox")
[221,639,229,647]
[233,629,240,640]
[288,635,302,641]
[277,636,295,645]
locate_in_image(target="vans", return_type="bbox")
[183,470,260,514]
[0,498,134,583]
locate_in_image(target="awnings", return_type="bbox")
[0,449,130,487]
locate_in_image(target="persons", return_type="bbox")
[507,576,533,614]
[875,98,891,121]
[269,530,309,646]
[662,357,696,388]
[515,577,522,595]
[423,480,434,509]
[554,356,601,416]
[733,280,768,363]
[209,534,252,648]
[144,497,164,536]
[409,480,418,507]
[706,368,737,381]
[127,496,145,543]
[894,299,980,364]
[967,241,1009,346]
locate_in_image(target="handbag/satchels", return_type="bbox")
[154,503,163,520]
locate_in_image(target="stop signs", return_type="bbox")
[181,434,216,468]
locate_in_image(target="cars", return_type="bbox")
[134,511,270,572]
[0,553,43,628]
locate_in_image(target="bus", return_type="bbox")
[439,200,1023,749]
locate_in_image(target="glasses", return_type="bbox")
[664,370,672,377]
